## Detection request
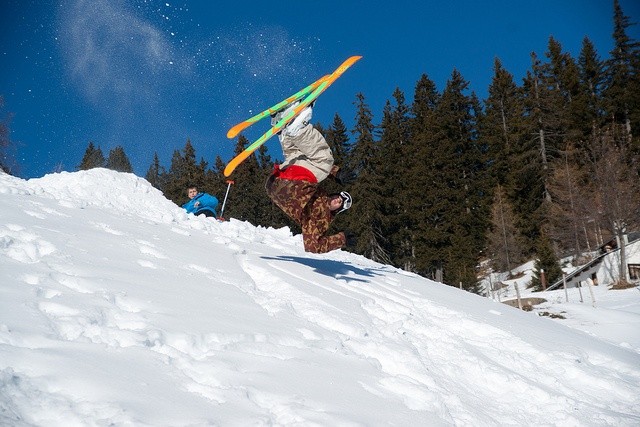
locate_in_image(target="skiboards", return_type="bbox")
[224,56,362,178]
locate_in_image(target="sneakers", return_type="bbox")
[286,107,312,136]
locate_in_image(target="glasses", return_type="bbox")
[337,191,352,215]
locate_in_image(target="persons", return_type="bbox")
[183,185,219,219]
[265,97,356,256]
